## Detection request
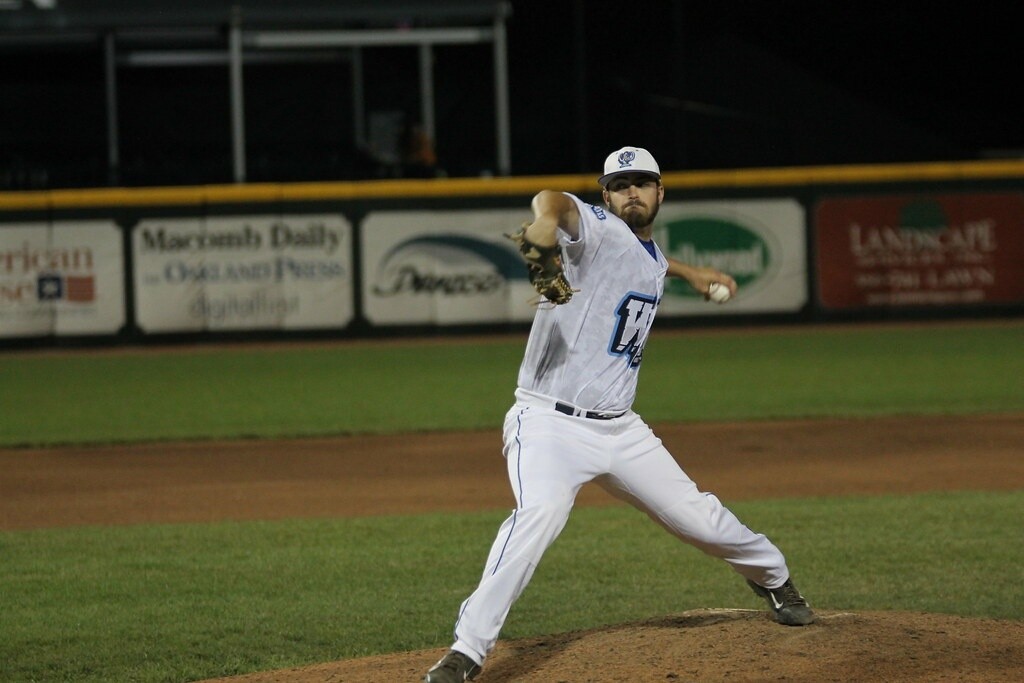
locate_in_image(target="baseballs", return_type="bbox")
[708,283,731,304]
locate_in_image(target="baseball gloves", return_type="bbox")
[502,221,581,311]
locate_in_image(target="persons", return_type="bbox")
[427,146,815,683]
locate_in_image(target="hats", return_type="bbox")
[596,146,661,190]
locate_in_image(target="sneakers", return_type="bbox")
[746,577,815,625]
[425,649,482,683]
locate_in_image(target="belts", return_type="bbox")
[557,402,626,420]
